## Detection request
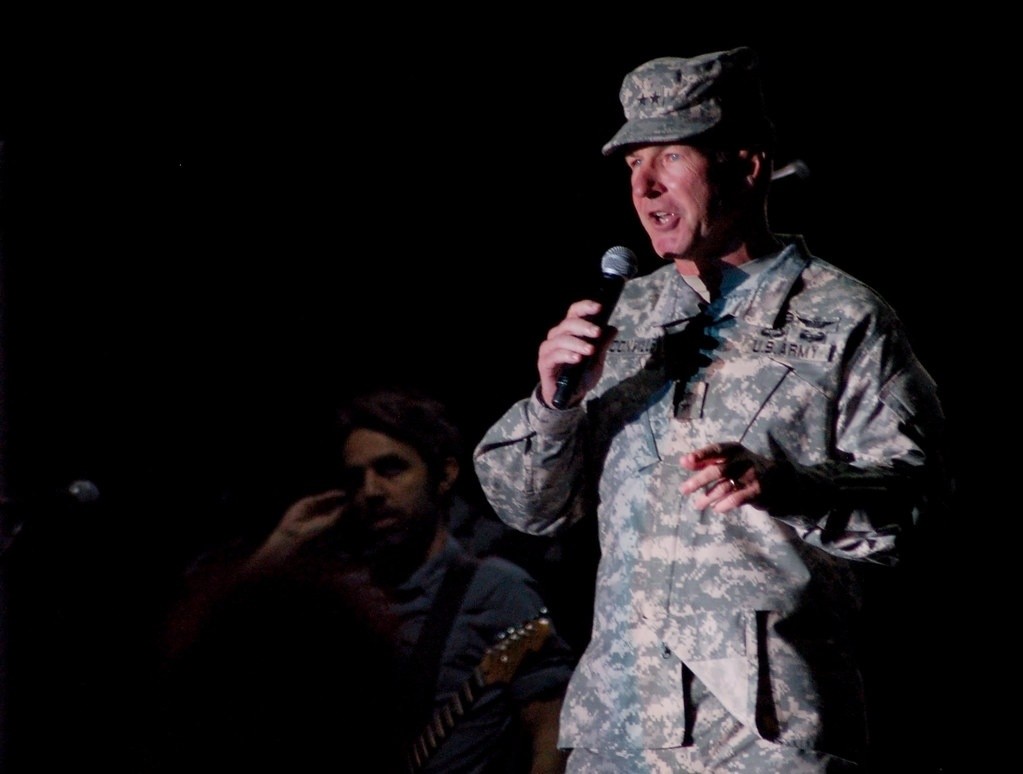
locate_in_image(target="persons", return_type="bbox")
[164,387,571,774]
[471,44,948,774]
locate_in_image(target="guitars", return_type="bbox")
[402,606,571,774]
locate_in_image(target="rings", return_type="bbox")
[729,479,735,487]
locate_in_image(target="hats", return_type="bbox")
[601,46,783,156]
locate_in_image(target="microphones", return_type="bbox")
[551,245,639,412]
[17,481,100,530]
[767,160,810,190]
